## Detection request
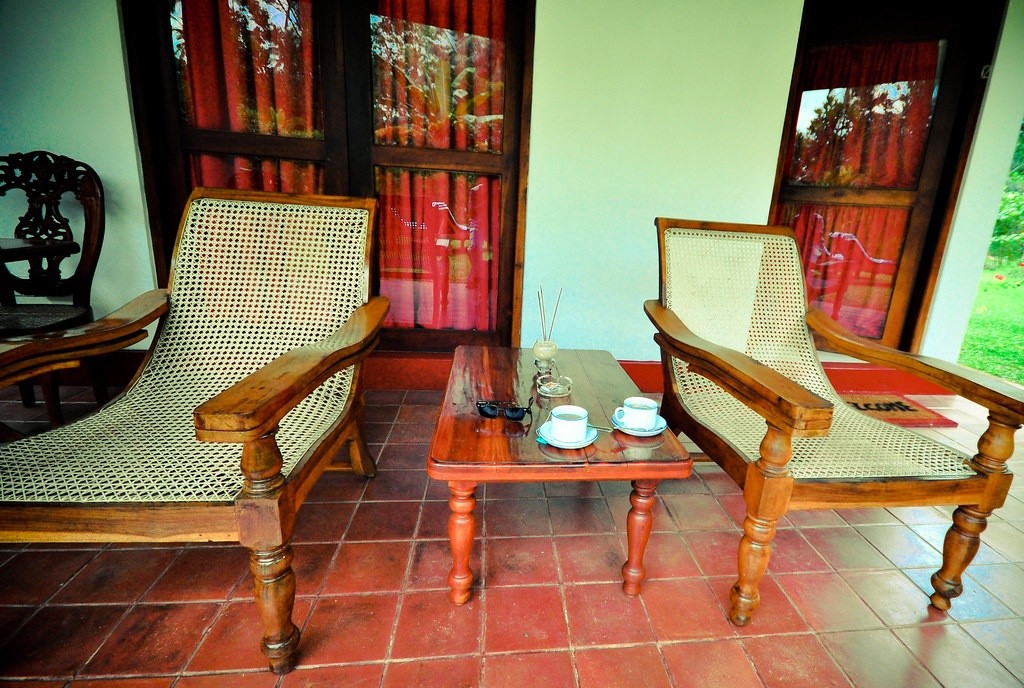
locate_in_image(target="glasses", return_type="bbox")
[473,422,530,439]
[476,397,533,421]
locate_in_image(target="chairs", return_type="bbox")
[0,150,105,408]
[643,217,1024,628]
[0,185,393,676]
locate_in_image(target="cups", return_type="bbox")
[614,397,658,430]
[550,404,589,443]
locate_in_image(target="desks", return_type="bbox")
[426,346,693,607]
[0,238,81,442]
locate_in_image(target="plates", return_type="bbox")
[539,421,598,449]
[612,411,667,437]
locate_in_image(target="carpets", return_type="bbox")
[836,390,958,428]
